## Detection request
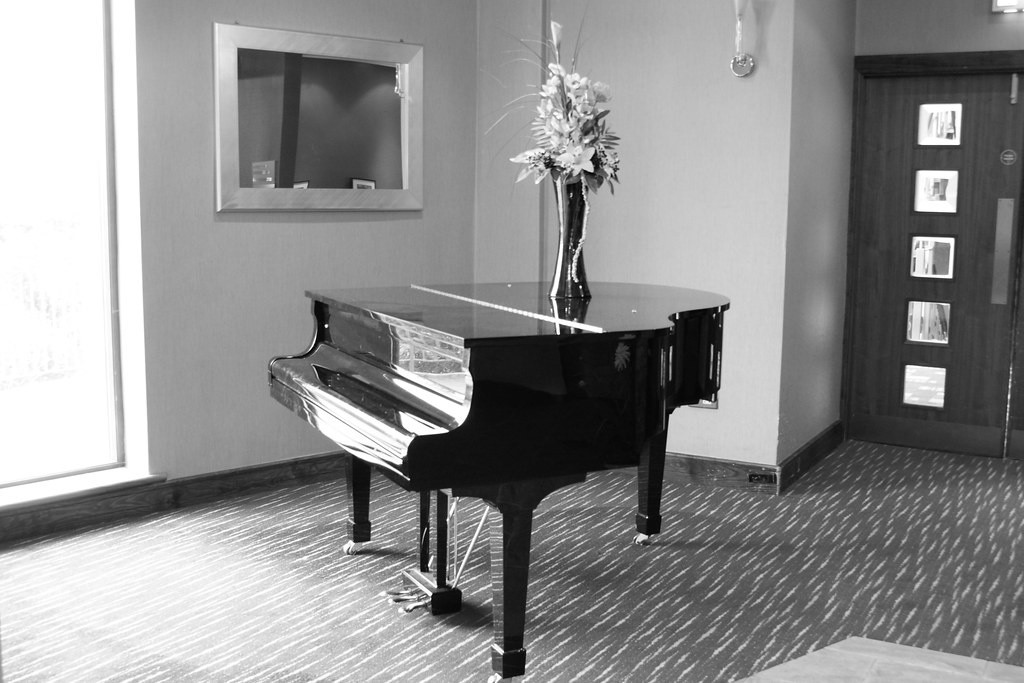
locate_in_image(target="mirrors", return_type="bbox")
[212,20,425,213]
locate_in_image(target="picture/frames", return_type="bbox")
[350,176,377,191]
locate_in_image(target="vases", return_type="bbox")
[546,167,603,301]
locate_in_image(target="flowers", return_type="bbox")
[480,8,624,193]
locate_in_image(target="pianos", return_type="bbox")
[266,280,732,683]
